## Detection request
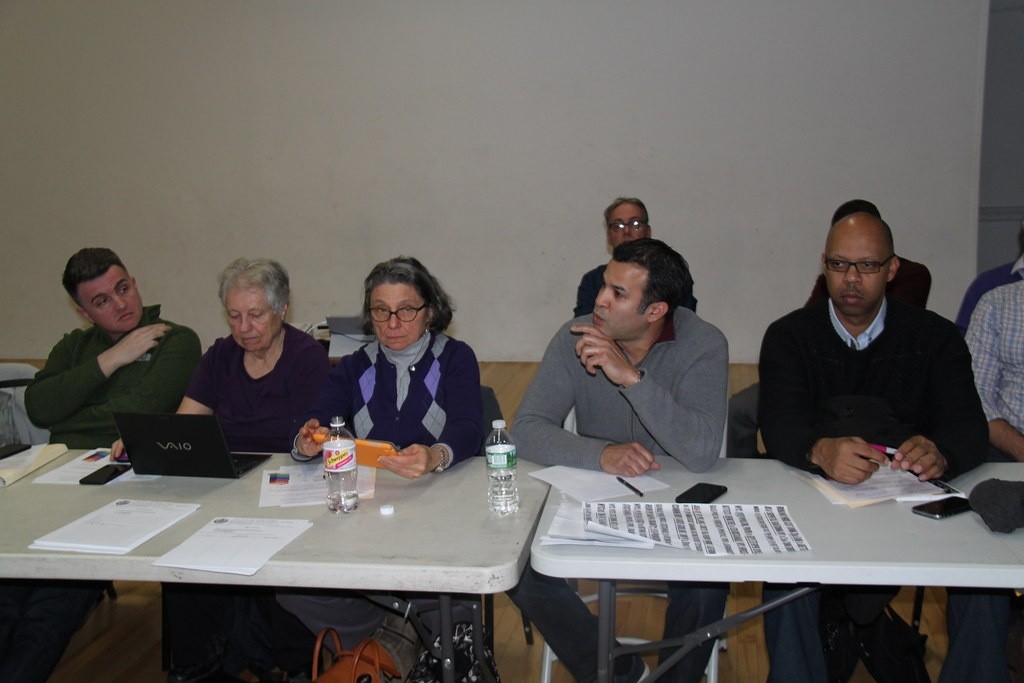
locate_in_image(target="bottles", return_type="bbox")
[320,417,359,514]
[484,419,519,517]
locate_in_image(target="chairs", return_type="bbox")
[0,363,117,604]
[542,385,728,683]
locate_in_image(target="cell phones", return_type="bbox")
[79,464,131,485]
[676,483,727,503]
[912,496,972,519]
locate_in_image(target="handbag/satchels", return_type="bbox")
[848,599,932,683]
[313,624,400,683]
[405,621,500,683]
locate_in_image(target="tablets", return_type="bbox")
[312,433,399,467]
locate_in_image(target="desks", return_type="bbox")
[530,458,1023,683]
[0,450,551,683]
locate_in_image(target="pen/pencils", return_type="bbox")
[617,476,643,498]
[867,444,898,454]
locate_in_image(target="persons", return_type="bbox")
[803,200,932,307]
[505,237,730,683]
[111,257,330,683]
[956,216,1024,327]
[939,258,1023,683]
[573,197,698,317]
[0,248,203,683]
[276,254,484,658]
[759,212,988,683]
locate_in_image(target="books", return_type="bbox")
[0,443,68,487]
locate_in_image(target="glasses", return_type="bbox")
[368,304,427,321]
[606,220,645,231]
[823,252,895,274]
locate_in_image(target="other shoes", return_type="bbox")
[168,655,218,683]
[617,652,653,683]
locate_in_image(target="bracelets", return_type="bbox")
[432,447,445,473]
[638,371,644,381]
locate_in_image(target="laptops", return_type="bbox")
[112,411,272,478]
